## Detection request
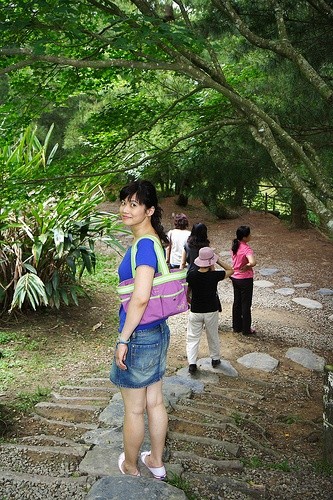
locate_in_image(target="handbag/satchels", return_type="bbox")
[166,230,173,263]
[116,234,188,325]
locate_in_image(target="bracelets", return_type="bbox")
[115,334,130,349]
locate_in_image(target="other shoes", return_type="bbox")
[119,452,141,476]
[189,364,197,374]
[212,359,221,368]
[242,330,255,335]
[141,451,166,479]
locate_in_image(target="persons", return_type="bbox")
[185,247,234,374]
[178,222,215,272]
[110,179,170,480]
[165,212,191,270]
[229,225,256,335]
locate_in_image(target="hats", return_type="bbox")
[194,247,218,267]
